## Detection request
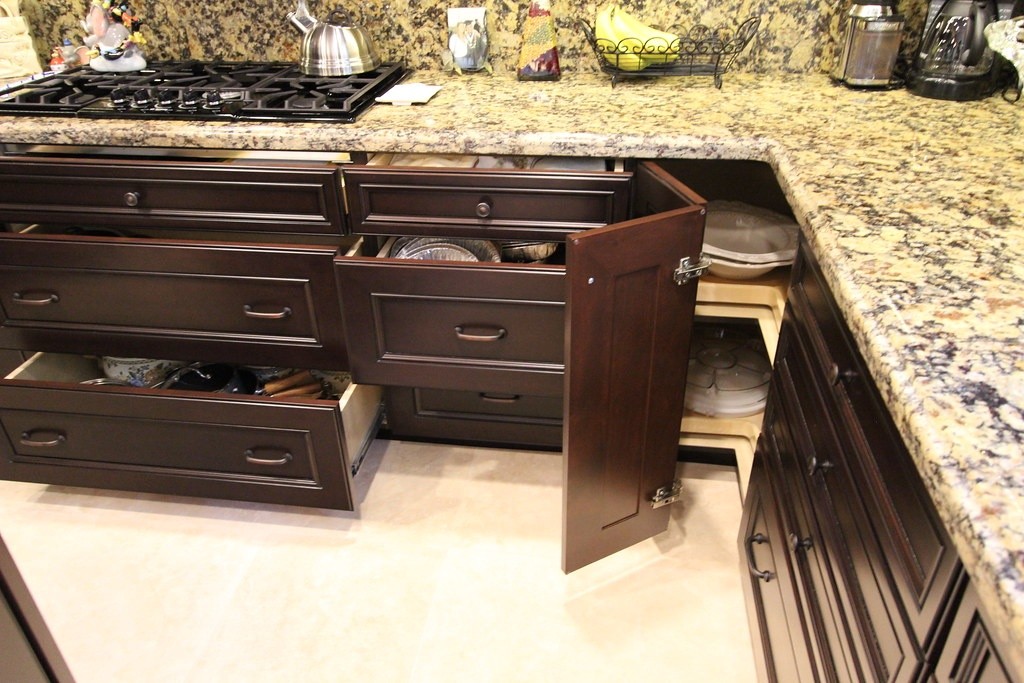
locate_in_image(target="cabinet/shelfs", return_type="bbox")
[0,142,1024,683]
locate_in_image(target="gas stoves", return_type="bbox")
[0,61,415,123]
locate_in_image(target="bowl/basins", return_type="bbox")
[702,254,794,280]
[242,365,294,384]
[103,356,185,386]
[311,369,352,395]
[161,361,237,394]
[701,200,799,264]
[80,378,138,387]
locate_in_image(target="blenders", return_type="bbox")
[905,0,1002,101]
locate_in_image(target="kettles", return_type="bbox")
[286,8,380,76]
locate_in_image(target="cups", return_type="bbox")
[845,15,906,86]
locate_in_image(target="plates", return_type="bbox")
[389,235,559,264]
[531,156,607,171]
